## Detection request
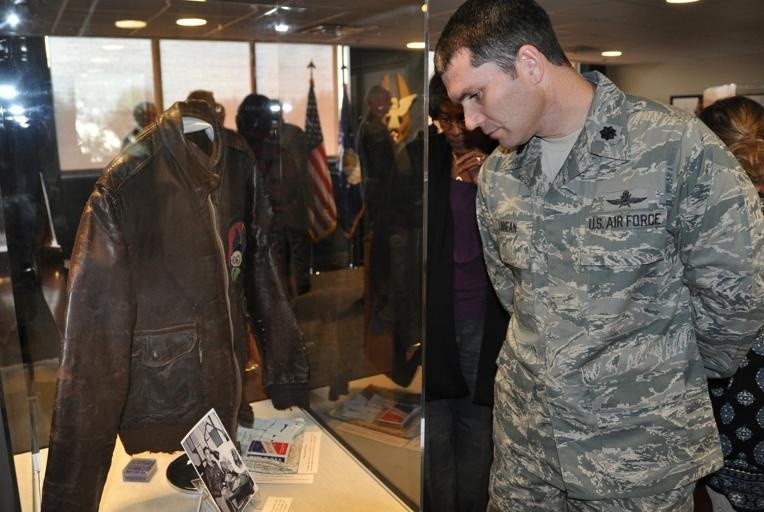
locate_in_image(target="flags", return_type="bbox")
[334,84,367,242]
[304,81,338,244]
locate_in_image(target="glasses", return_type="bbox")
[431,113,467,132]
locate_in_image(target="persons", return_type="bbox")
[433,1,764,512]
[696,95,764,512]
[257,96,311,302]
[382,72,509,512]
[119,101,158,153]
[353,84,398,314]
[184,89,225,126]
[202,447,238,512]
